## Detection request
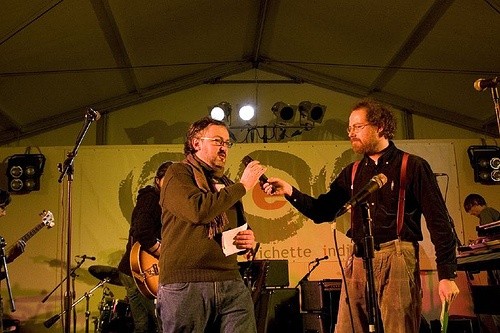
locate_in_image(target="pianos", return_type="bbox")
[456,220,500,314]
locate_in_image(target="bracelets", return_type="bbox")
[152,245,161,254]
[449,277,456,281]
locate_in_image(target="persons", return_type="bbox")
[124,118,258,333]
[0,190,25,333]
[464,194,500,333]
[258,101,460,333]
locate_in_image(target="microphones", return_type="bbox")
[474,77,500,91]
[242,156,276,193]
[336,173,387,217]
[86,107,100,120]
[106,287,114,297]
[77,256,96,260]
[434,173,447,176]
[310,256,329,263]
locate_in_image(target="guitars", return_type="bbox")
[0,209,55,281]
[251,260,270,303]
[130,238,161,300]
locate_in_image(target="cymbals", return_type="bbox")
[88,265,123,286]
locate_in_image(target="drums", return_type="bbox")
[96,297,133,333]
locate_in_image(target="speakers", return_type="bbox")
[302,280,343,313]
[430,318,480,333]
[302,313,337,333]
[253,288,302,333]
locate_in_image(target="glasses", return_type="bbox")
[348,123,376,131]
[196,137,233,147]
